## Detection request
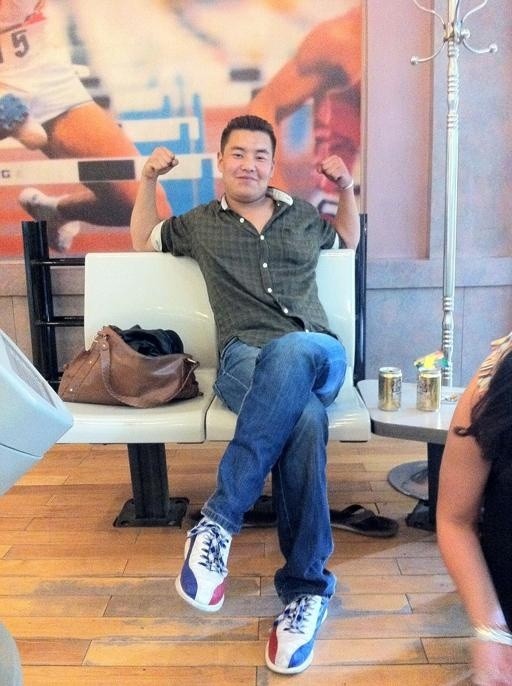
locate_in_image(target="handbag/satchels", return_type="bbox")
[56,324,202,409]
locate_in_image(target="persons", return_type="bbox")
[130,114,361,676]
[244,2,365,378]
[436,342,511,686]
[0,0,174,253]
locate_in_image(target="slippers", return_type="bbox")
[328,503,400,540]
[189,506,280,529]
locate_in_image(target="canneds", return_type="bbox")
[379,366,403,412]
[417,365,442,411]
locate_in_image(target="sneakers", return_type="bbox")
[174,515,234,613]
[264,592,331,675]
[17,188,82,253]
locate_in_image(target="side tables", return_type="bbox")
[358,380,478,530]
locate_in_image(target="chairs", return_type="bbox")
[207,248,371,529]
[51,250,219,529]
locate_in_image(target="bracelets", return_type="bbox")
[337,177,353,192]
